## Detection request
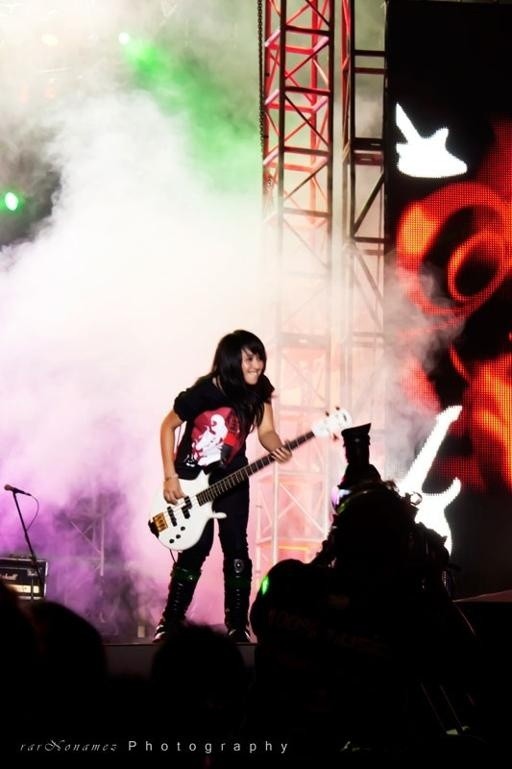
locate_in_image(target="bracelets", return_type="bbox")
[164,474,180,482]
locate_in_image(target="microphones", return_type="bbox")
[5,484,30,497]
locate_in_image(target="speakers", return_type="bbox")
[0,557,48,609]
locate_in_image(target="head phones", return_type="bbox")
[327,483,416,556]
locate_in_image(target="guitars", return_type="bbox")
[148,406,354,551]
[395,405,463,556]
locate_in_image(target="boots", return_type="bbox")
[223,557,252,641]
[153,562,203,642]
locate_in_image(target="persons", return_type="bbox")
[149,325,294,644]
[1,481,511,769]
